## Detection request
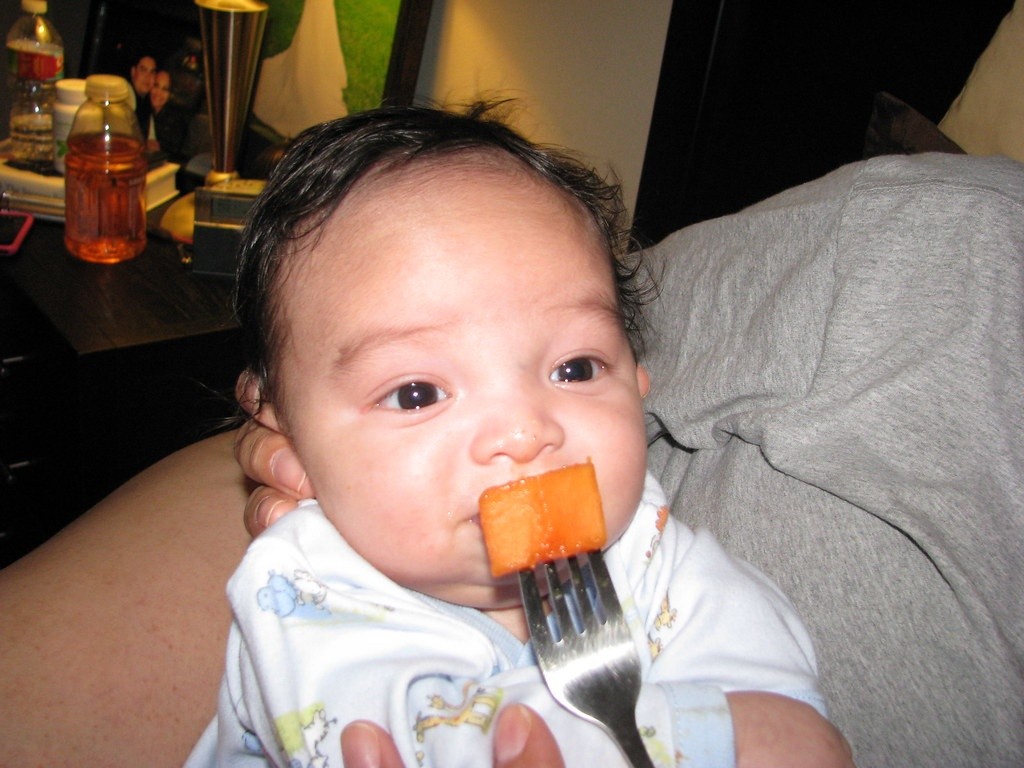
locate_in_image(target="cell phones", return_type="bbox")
[0,211,34,256]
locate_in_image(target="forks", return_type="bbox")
[520,547,654,768]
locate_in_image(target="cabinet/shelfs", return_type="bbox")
[0,142,245,572]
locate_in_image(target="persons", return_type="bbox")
[129,55,197,191]
[0,152,1024,768]
[179,107,854,768]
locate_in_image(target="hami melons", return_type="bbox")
[480,461,607,577]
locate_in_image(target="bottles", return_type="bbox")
[64,74,148,264]
[55,78,89,173]
[5,0,64,167]
